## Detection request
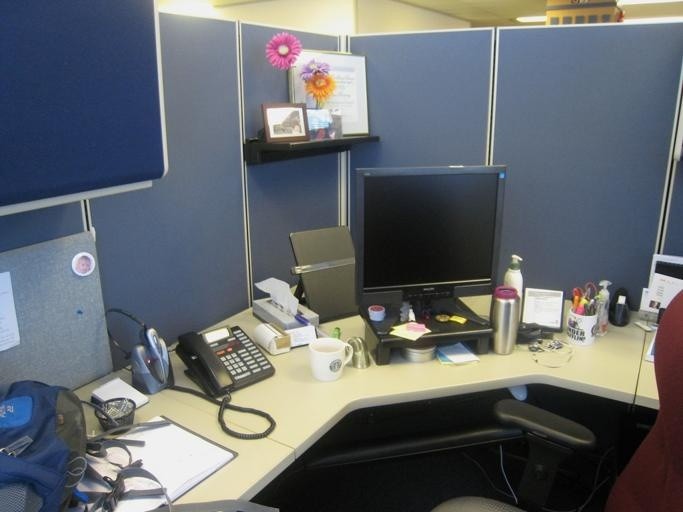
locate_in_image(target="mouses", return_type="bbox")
[506,386,530,402]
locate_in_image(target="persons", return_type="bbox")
[77,257,91,274]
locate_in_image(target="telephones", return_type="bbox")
[176,326,275,398]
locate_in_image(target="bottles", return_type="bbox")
[489,286,521,356]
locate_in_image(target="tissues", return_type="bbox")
[252,277,320,333]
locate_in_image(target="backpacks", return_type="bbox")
[0,380,280,512]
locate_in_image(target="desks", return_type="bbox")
[73,282,663,511]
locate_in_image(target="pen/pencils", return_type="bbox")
[455,313,487,326]
[295,313,329,337]
[574,291,604,315]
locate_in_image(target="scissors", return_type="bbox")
[578,283,597,298]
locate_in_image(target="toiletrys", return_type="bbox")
[503,253,523,321]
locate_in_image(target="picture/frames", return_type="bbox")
[259,101,311,144]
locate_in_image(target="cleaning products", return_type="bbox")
[599,279,612,335]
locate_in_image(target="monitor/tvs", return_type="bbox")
[353,165,508,335]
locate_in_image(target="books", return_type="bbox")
[77,413,238,512]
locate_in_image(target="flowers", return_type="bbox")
[264,31,337,110]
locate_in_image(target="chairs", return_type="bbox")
[428,290,683,512]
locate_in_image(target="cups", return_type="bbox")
[566,307,598,347]
[308,338,355,385]
